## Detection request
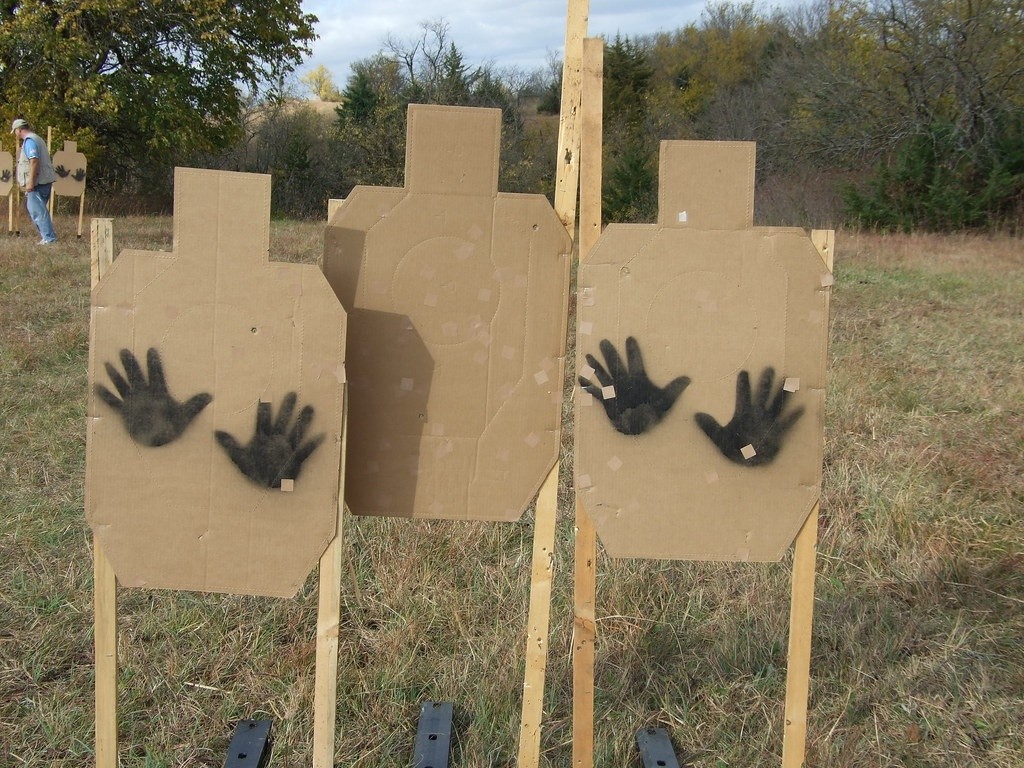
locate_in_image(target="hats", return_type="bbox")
[10,119,28,134]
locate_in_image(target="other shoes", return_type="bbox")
[37,238,58,246]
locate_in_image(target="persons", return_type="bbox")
[10,119,57,246]
